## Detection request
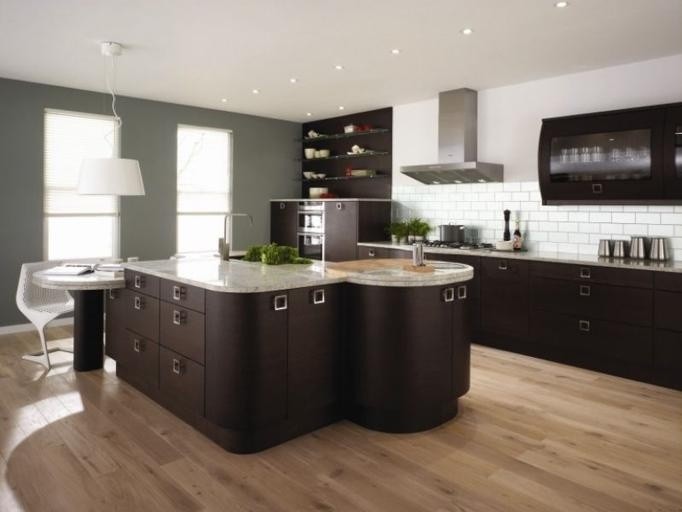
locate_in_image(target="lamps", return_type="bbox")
[75,41,147,198]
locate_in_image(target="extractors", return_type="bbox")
[399,88,504,186]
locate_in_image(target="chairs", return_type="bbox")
[15,259,77,370]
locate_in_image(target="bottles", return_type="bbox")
[513,219,523,252]
[560,146,649,165]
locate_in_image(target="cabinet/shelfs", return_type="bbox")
[426,253,682,395]
[105,269,470,455]
[293,128,389,182]
[271,202,392,263]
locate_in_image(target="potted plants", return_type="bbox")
[383,217,431,244]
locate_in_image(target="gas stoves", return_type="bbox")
[411,238,495,250]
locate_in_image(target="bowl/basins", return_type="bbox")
[302,170,328,179]
[303,147,331,159]
[495,241,512,250]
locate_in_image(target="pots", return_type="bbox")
[437,223,466,244]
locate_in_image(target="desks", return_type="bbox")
[28,265,128,372]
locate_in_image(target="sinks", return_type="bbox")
[227,254,248,262]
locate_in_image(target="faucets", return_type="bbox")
[223,211,254,261]
